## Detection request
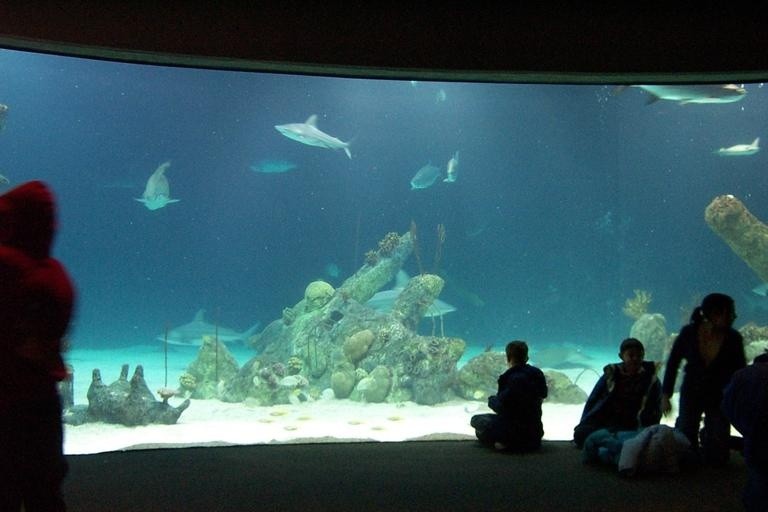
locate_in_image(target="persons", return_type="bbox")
[470,340,547,453]
[1,178,77,511]
[573,337,664,446]
[719,352,768,511]
[661,293,748,467]
